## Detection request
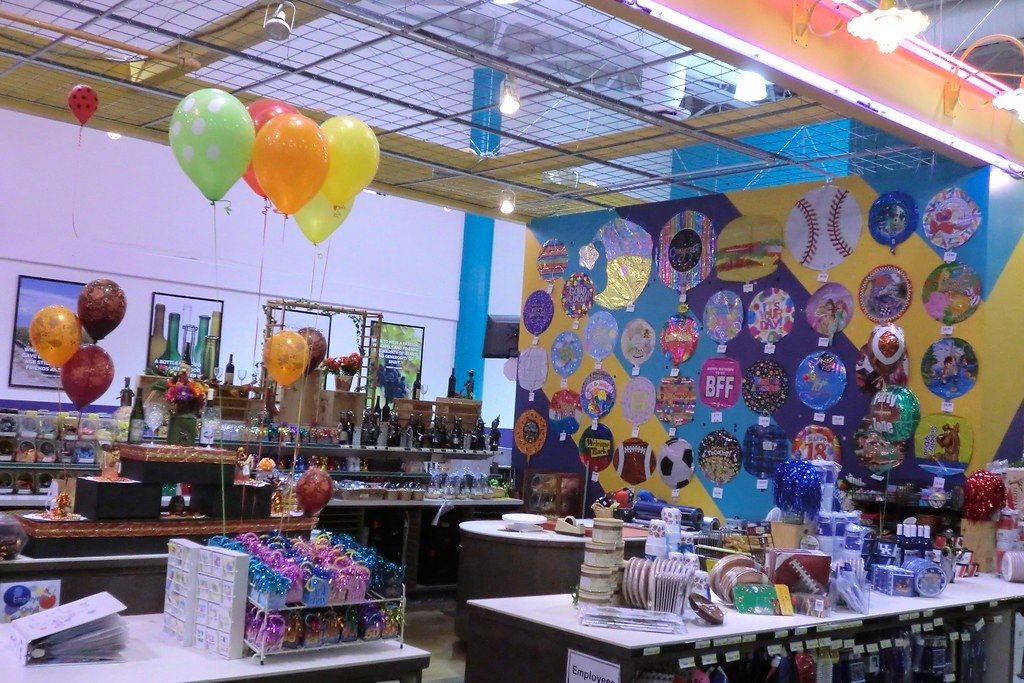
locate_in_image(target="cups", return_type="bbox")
[644,508,708,598]
[578,518,628,609]
[332,488,425,503]
[810,462,863,564]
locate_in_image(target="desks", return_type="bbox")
[463,571,1024,683]
[457,520,663,640]
[0,618,430,683]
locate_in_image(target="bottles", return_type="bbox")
[895,525,932,566]
[199,388,215,446]
[120,377,144,444]
[996,511,1024,574]
[375,395,390,423]
[148,304,222,382]
[448,368,456,398]
[225,354,234,385]
[339,411,463,449]
[413,373,421,400]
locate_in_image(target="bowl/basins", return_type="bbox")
[502,513,547,532]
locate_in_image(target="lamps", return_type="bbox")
[499,79,522,114]
[500,190,517,213]
[264,0,295,39]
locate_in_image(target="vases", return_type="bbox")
[335,374,353,391]
[167,400,199,447]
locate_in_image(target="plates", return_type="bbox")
[425,492,494,499]
[622,555,772,623]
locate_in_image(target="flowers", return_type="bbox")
[151,372,209,410]
[321,353,366,377]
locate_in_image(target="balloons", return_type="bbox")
[29,304,83,368]
[296,469,333,515]
[61,344,115,408]
[69,84,99,124]
[869,323,907,379]
[169,88,256,202]
[297,327,326,377]
[78,279,127,344]
[870,385,921,445]
[321,116,380,209]
[241,100,301,198]
[253,114,330,216]
[293,191,355,243]
[263,330,310,388]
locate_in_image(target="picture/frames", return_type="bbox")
[9,275,103,392]
[364,319,425,411]
[144,292,224,383]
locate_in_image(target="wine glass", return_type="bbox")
[203,404,221,450]
[214,367,222,381]
[143,402,165,445]
[238,370,247,385]
